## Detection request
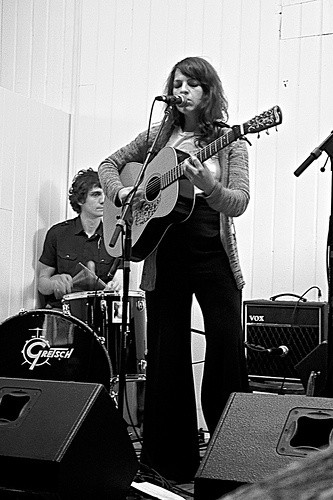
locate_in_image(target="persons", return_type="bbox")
[37,167,124,312]
[98,58,253,485]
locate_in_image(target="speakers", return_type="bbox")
[0,377,139,500]
[194,392,333,500]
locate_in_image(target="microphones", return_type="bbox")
[156,94,187,107]
[268,345,288,357]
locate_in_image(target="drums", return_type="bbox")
[61,290,149,381]
[0,304,120,396]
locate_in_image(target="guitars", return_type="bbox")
[102,106,284,263]
